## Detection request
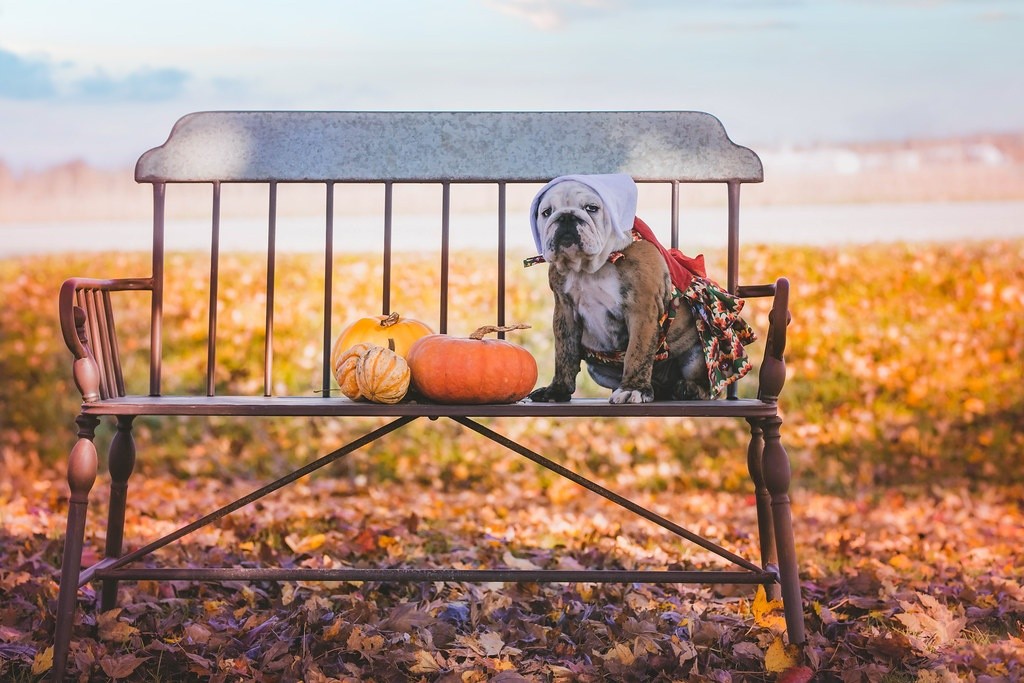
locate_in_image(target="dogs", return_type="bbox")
[527,180,723,406]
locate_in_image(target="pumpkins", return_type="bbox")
[331,312,436,386]
[335,343,370,402]
[407,323,539,404]
[356,338,411,403]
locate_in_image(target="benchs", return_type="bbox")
[53,111,811,683]
